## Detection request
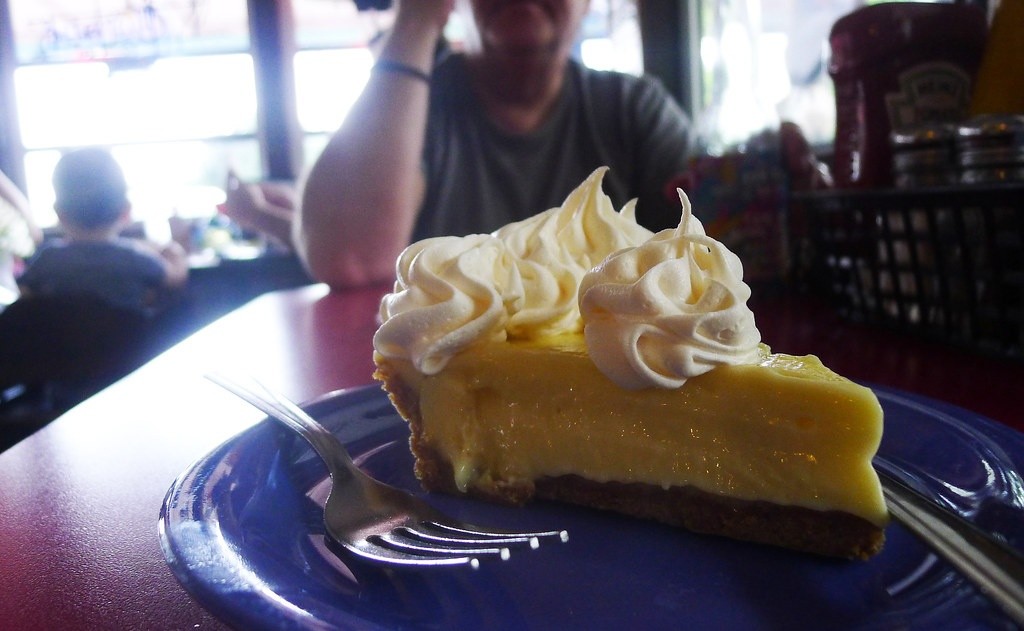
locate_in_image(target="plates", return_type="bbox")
[158,375,1024,631]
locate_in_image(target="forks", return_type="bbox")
[201,366,568,571]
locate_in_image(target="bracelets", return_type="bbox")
[370,60,431,85]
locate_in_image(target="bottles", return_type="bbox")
[881,131,962,319]
[955,113,1024,299]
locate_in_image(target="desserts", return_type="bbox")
[371,164,888,563]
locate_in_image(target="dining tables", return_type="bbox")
[0,277,1024,631]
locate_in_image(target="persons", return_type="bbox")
[26,146,190,426]
[216,26,454,253]
[0,167,43,405]
[300,0,700,286]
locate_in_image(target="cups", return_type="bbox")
[169,216,206,256]
